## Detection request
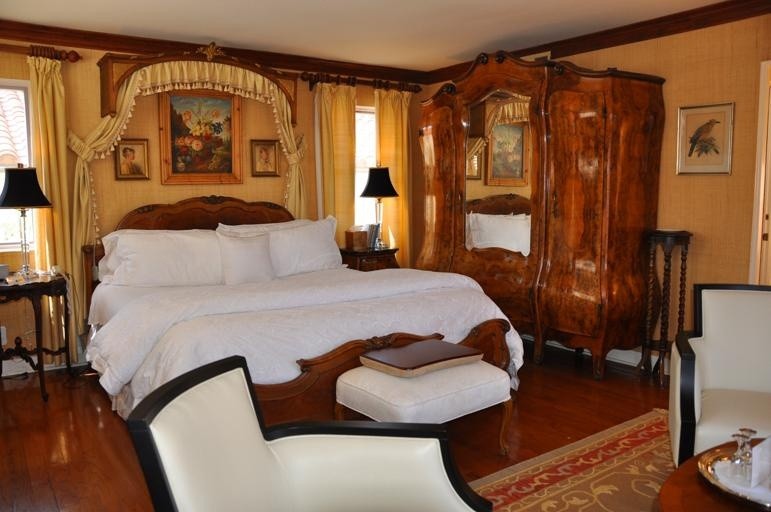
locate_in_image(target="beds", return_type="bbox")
[465,193,531,214]
[82,195,510,428]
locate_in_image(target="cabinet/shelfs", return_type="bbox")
[415,50,665,381]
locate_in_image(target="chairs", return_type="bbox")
[669,284,771,469]
[127,355,492,512]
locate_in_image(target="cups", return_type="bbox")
[0,264,10,280]
[51,265,61,276]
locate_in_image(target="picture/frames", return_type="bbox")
[676,101,734,175]
[115,138,149,180]
[484,117,529,186]
[158,88,244,185]
[250,140,279,177]
[466,152,482,180]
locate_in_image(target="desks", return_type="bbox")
[0,273,74,402]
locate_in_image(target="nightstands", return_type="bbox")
[340,247,399,272]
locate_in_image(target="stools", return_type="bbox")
[334,360,513,456]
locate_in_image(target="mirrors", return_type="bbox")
[462,87,536,258]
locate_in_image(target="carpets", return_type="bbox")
[467,408,674,512]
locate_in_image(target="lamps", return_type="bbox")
[0,164,53,280]
[360,168,398,248]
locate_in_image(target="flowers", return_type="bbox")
[175,109,231,172]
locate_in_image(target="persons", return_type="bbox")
[257,147,275,172]
[120,147,143,176]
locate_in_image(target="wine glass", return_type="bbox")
[728,425,757,481]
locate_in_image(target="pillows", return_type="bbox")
[466,210,531,256]
[98,215,343,285]
